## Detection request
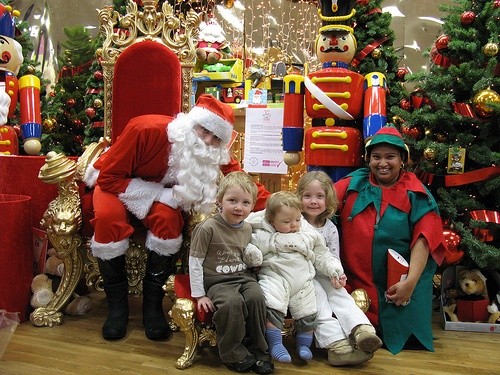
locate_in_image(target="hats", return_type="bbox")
[364,122,410,163]
[188,94,235,143]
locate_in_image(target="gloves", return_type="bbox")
[154,187,179,208]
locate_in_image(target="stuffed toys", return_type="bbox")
[196,10,230,64]
[441,269,500,324]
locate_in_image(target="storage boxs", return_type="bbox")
[442,294,500,333]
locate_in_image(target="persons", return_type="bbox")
[251,190,344,362]
[282,0,387,183]
[86,95,275,341]
[296,170,382,365]
[188,171,275,374]
[332,122,449,356]
[0,3,41,156]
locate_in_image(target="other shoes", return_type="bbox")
[351,325,382,353]
[328,340,374,365]
[223,356,257,373]
[251,360,274,375]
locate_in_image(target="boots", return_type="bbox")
[142,229,184,342]
[91,232,131,341]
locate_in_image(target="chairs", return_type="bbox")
[32,0,370,369]
